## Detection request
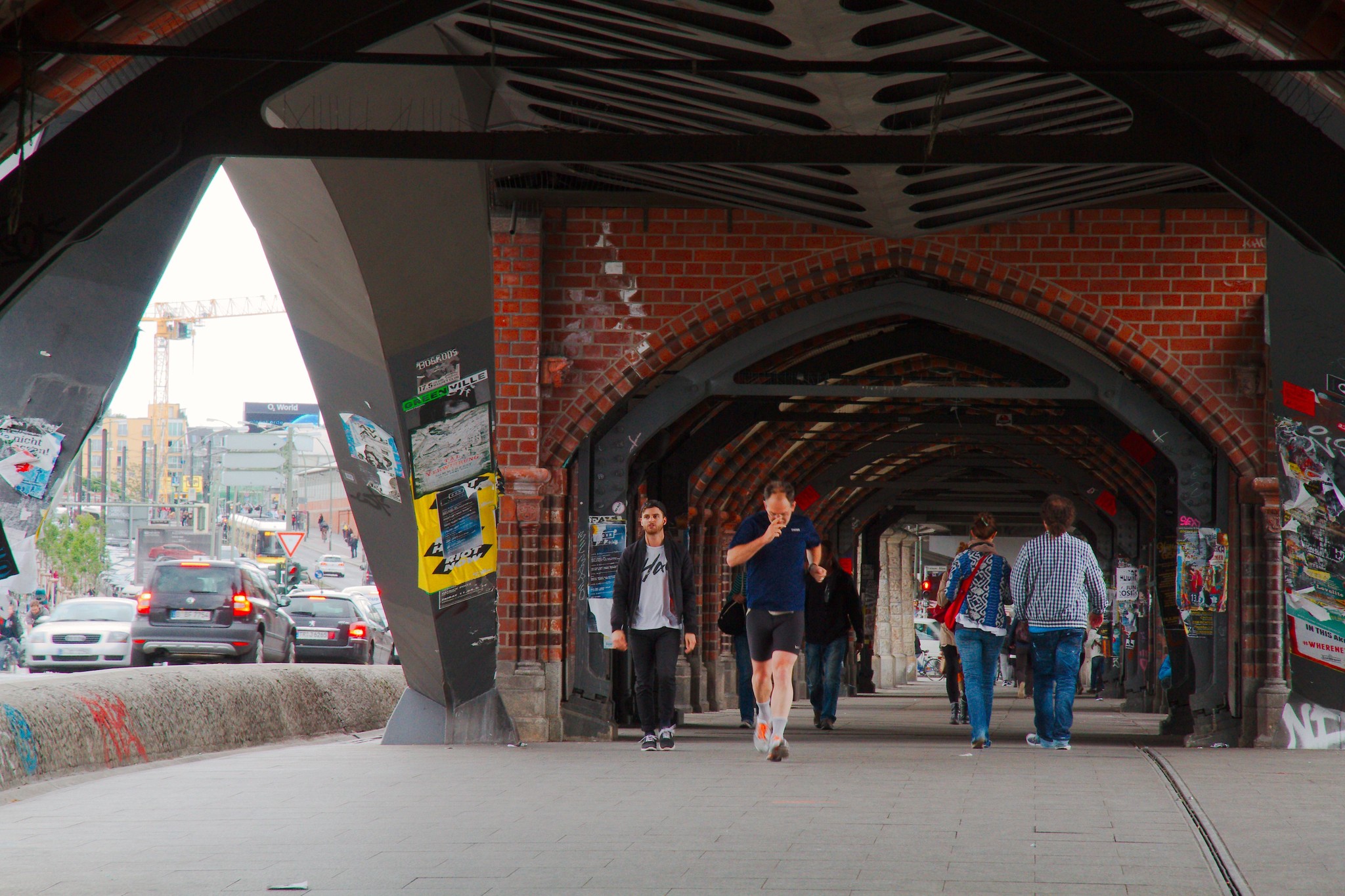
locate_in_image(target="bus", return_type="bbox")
[227,512,287,570]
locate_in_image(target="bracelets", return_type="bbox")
[809,563,817,572]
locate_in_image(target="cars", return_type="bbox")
[25,598,137,673]
[215,514,230,524]
[148,543,206,561]
[914,618,941,675]
[97,545,144,598]
[315,554,345,578]
[360,561,375,586]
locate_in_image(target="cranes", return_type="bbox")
[141,295,287,503]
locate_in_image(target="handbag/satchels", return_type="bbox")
[717,598,747,636]
[933,600,951,623]
[1015,620,1028,643]
[945,554,988,632]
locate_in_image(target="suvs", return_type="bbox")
[279,588,395,665]
[131,558,298,667]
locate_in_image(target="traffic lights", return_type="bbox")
[923,581,930,599]
[268,562,308,586]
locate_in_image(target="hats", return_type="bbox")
[640,500,666,515]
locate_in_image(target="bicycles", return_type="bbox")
[917,650,944,681]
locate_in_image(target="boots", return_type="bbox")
[960,698,970,724]
[949,702,962,725]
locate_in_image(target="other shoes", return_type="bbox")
[1076,685,1103,700]
[982,744,988,748]
[993,680,1033,699]
[813,714,822,728]
[821,719,834,730]
[740,719,754,729]
[970,737,984,749]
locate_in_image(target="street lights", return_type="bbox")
[155,422,332,552]
[146,447,154,493]
[106,447,113,495]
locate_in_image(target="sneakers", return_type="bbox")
[1055,742,1071,750]
[766,734,790,762]
[754,714,772,752]
[1026,733,1042,746]
[658,724,676,750]
[637,734,658,751]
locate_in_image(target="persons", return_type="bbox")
[1158,654,1172,715]
[937,542,970,724]
[1084,625,1105,694]
[1000,616,1012,687]
[1011,613,1033,699]
[727,480,827,762]
[729,563,759,729]
[804,540,864,730]
[1076,630,1088,695]
[610,500,696,751]
[1010,494,1109,750]
[944,513,1012,749]
[915,628,925,673]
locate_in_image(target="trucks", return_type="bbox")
[342,585,400,665]
[51,505,108,534]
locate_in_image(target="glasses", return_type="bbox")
[765,507,792,519]
